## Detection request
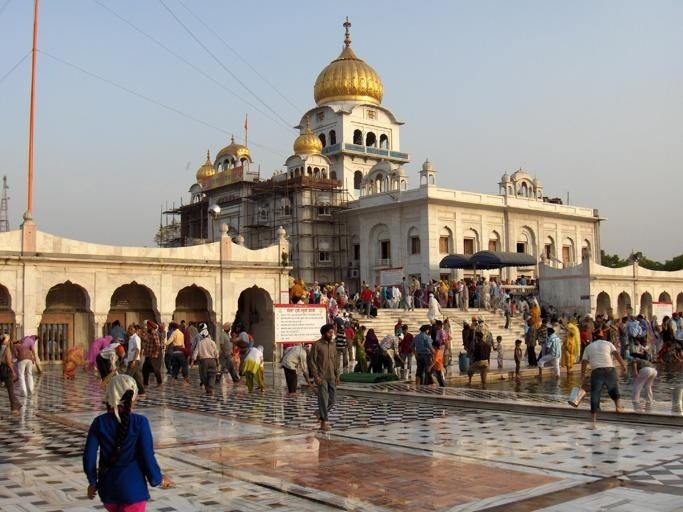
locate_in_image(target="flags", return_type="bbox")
[243,118,247,129]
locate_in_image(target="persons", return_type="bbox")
[81,375,176,512]
[581,329,627,430]
[309,324,340,432]
[0,334,39,413]
[328,297,405,374]
[281,343,312,395]
[64,344,86,378]
[428,292,443,326]
[626,358,657,405]
[460,315,503,387]
[289,274,539,311]
[394,318,453,388]
[85,320,267,395]
[502,296,683,382]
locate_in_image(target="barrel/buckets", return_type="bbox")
[569,385,587,408]
[216,370,227,383]
[400,369,410,380]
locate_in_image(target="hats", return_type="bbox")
[472,317,477,321]
[116,344,125,353]
[429,293,434,297]
